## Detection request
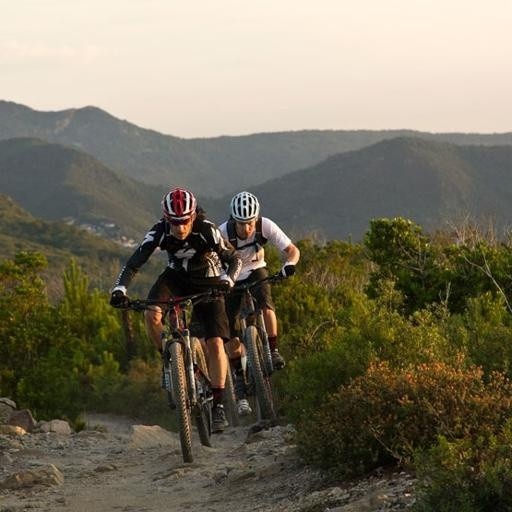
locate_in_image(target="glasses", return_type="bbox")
[171,219,190,227]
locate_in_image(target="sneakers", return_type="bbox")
[235,397,254,417]
[270,350,286,368]
[209,404,229,434]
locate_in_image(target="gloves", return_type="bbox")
[209,276,236,298]
[279,262,298,279]
[108,288,132,309]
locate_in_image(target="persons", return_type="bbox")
[108,188,242,430]
[218,192,301,418]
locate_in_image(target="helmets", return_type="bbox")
[160,186,196,221]
[229,190,260,225]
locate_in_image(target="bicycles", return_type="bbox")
[113,271,283,463]
